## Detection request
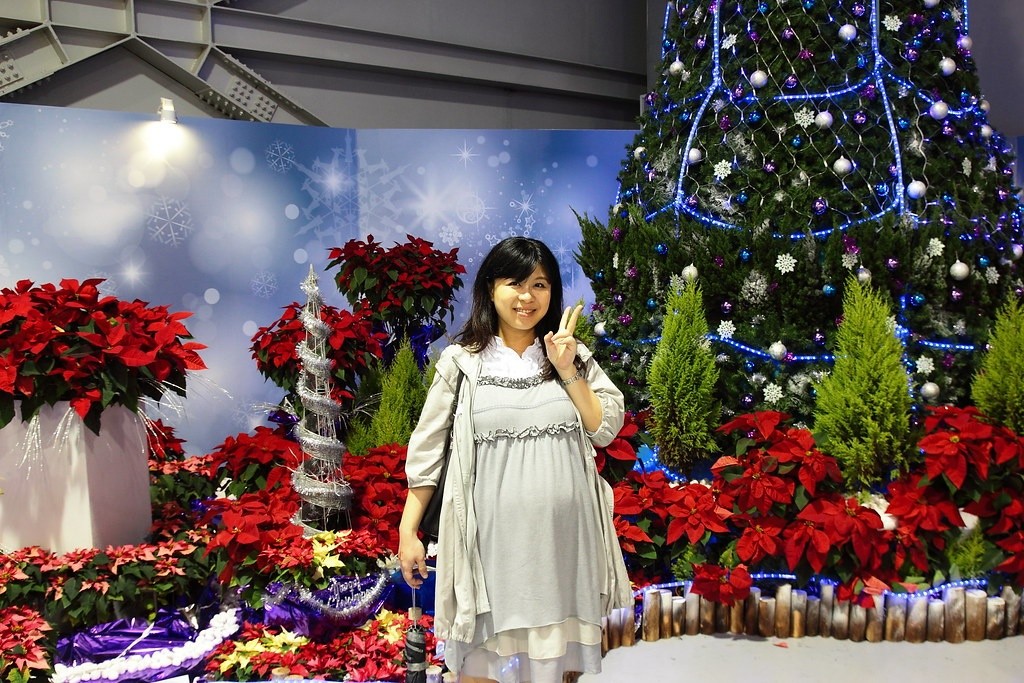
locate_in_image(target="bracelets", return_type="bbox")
[563,371,582,386]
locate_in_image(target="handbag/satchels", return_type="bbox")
[419,370,465,543]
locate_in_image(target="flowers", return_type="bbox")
[324,233,466,326]
[249,301,387,416]
[1,280,232,474]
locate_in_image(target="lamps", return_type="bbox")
[158,97,177,126]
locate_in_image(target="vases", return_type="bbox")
[268,394,347,446]
[373,321,444,377]
[1,397,154,553]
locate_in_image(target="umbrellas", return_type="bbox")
[405,606,427,683]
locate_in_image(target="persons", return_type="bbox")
[396,237,637,682]
